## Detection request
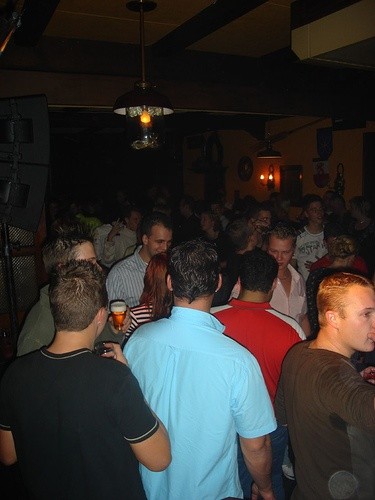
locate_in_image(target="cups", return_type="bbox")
[96,341,116,359]
[363,367,375,381]
[111,301,126,332]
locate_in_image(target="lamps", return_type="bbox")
[112,0,175,151]
[259,165,275,191]
[255,120,283,158]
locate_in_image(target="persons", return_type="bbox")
[0,188,375,499]
